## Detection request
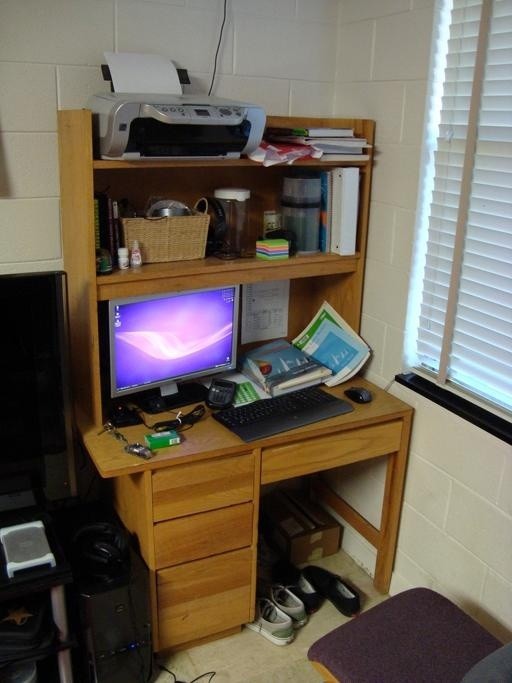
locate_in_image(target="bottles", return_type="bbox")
[213,187,252,261]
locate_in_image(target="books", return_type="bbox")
[246,126,371,259]
[91,190,126,269]
[200,299,371,408]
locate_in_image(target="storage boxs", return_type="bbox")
[259,487,341,565]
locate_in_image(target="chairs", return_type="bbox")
[307,584,512,683]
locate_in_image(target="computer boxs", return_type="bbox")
[52,518,152,682]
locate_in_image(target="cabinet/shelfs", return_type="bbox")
[51,108,377,424]
[0,466,78,682]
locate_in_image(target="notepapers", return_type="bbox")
[256,239,289,261]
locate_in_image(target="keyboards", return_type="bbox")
[211,386,354,444]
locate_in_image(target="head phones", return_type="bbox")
[68,519,134,576]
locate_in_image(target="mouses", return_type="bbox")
[343,385,372,405]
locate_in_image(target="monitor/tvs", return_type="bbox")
[107,284,241,414]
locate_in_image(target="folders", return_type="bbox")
[330,166,360,256]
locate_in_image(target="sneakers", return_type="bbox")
[303,566,360,617]
[262,581,307,628]
[245,597,294,645]
[277,562,319,612]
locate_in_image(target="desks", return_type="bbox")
[66,373,415,656]
